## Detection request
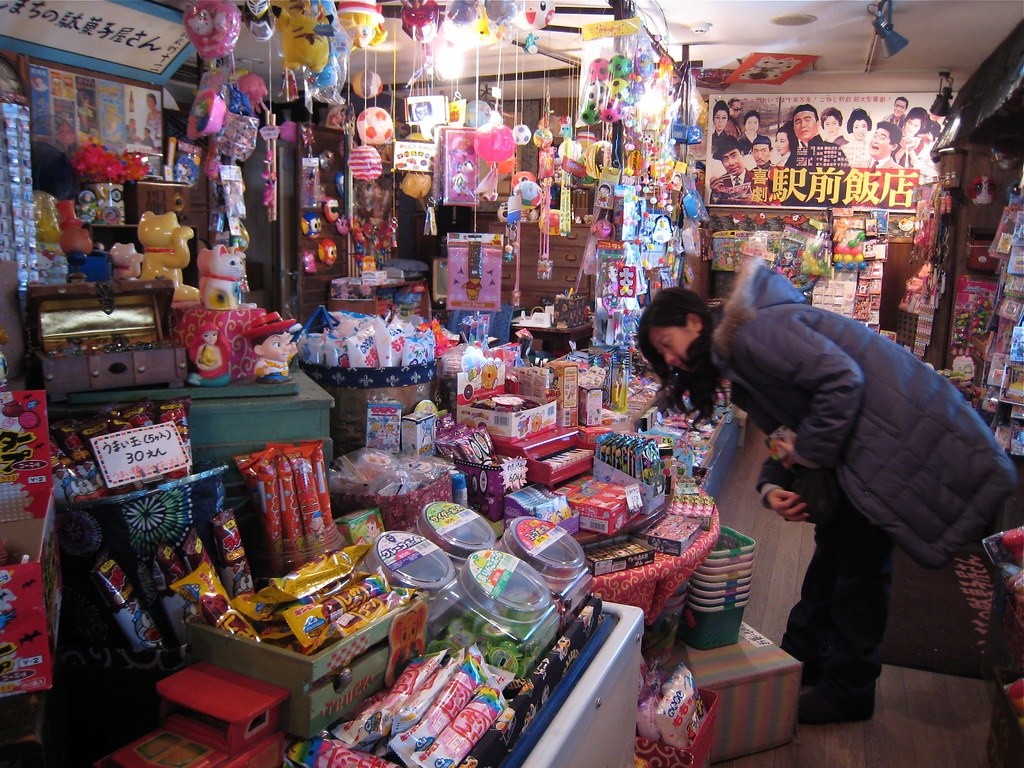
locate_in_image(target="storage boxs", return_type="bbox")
[362,271,388,278]
[578,384,603,426]
[594,456,667,515]
[362,279,384,285]
[667,496,716,531]
[632,687,719,768]
[26,276,188,394]
[580,535,657,577]
[457,345,557,443]
[553,475,641,536]
[544,361,579,427]
[185,578,430,740]
[645,514,703,557]
[401,410,437,459]
[334,508,386,546]
[366,399,401,455]
[640,413,651,431]
[451,457,528,522]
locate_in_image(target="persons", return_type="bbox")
[187,324,232,387]
[242,311,299,384]
[637,256,1018,723]
[711,96,941,206]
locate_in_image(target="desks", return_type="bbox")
[45,367,337,540]
[510,319,592,358]
[541,473,721,624]
[489,221,595,315]
[327,279,432,324]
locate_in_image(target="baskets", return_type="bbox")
[679,524,756,649]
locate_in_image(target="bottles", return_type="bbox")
[357,501,593,678]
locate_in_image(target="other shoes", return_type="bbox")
[798,686,875,725]
[780,645,821,686]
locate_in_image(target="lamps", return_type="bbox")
[866,0,908,58]
[930,72,954,116]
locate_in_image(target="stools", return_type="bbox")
[661,620,804,763]
